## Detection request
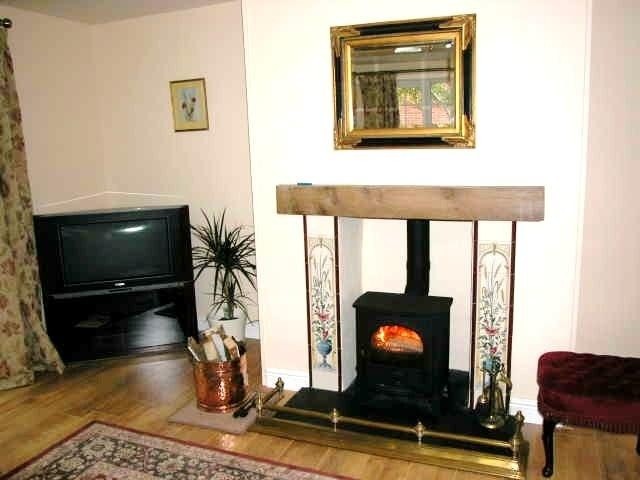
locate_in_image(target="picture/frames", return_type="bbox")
[169,78,209,132]
[329,14,476,149]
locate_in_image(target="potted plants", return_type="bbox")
[189,208,257,342]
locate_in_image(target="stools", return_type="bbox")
[537,352,640,477]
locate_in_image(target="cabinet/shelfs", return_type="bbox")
[42,280,197,366]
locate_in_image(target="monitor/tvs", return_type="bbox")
[55,214,176,292]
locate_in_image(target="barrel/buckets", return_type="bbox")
[191,345,250,414]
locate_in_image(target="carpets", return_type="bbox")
[167,393,284,434]
[0,420,359,480]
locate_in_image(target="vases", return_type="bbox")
[483,359,499,384]
[316,341,331,368]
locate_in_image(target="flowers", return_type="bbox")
[314,302,332,344]
[478,305,507,361]
